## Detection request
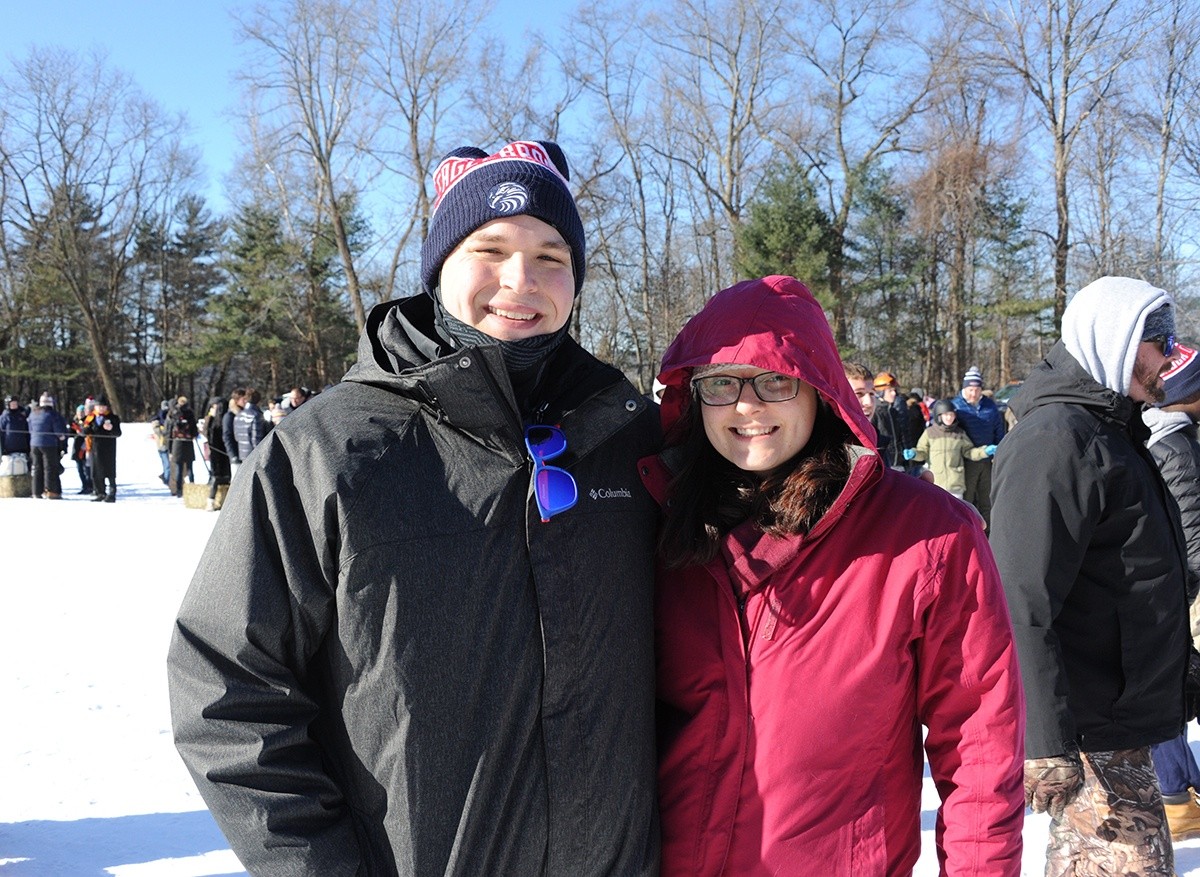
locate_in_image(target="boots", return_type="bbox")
[206,498,215,512]
[1161,787,1200,840]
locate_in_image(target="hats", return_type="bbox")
[962,366,984,388]
[85,396,95,406]
[40,392,54,408]
[1152,343,1200,408]
[419,140,590,296]
[1143,304,1175,341]
[692,363,754,383]
[160,397,224,410]
[94,395,108,406]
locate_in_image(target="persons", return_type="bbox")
[842,361,1010,530]
[988,274,1200,877]
[1140,341,1200,840]
[1,385,311,502]
[167,139,665,877]
[639,274,1027,877]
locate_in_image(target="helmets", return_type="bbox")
[934,400,955,417]
[872,376,895,391]
[4,395,18,410]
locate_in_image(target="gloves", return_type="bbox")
[904,449,916,460]
[1024,749,1085,816]
[985,445,998,456]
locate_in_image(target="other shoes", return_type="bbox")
[78,490,93,495]
[46,491,60,498]
[91,494,107,502]
[159,473,166,483]
[104,493,115,503]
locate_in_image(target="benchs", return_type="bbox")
[0,474,33,498]
[183,483,230,510]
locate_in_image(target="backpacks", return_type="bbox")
[172,412,192,440]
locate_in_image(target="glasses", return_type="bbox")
[524,423,579,523]
[692,371,801,406]
[1149,335,1175,357]
